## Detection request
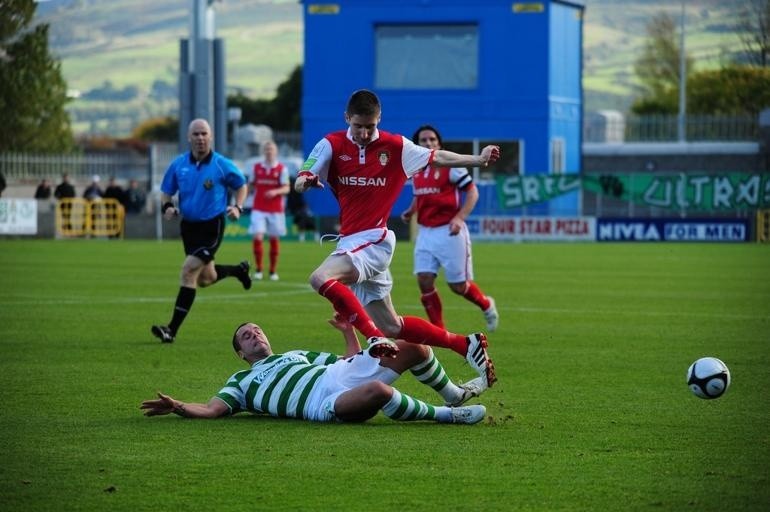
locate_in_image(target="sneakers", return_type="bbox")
[253,270,280,282]
[443,331,496,425]
[367,335,400,360]
[152,324,175,344]
[238,260,251,290]
[483,296,498,333]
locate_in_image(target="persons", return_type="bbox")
[400,126,501,333]
[150,118,251,343]
[293,90,502,388]
[140,310,490,426]
[245,138,291,281]
[284,172,321,244]
[31,169,148,241]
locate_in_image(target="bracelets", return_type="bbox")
[161,202,173,213]
[234,204,244,214]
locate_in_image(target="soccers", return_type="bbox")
[686,357,729,400]
